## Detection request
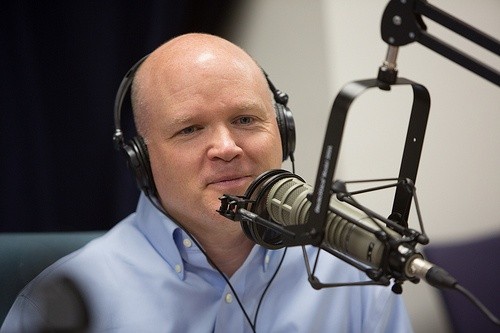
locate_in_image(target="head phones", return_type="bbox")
[113,54,296,197]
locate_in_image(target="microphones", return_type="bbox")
[242,170,457,289]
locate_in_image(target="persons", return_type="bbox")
[0,32,412,332]
